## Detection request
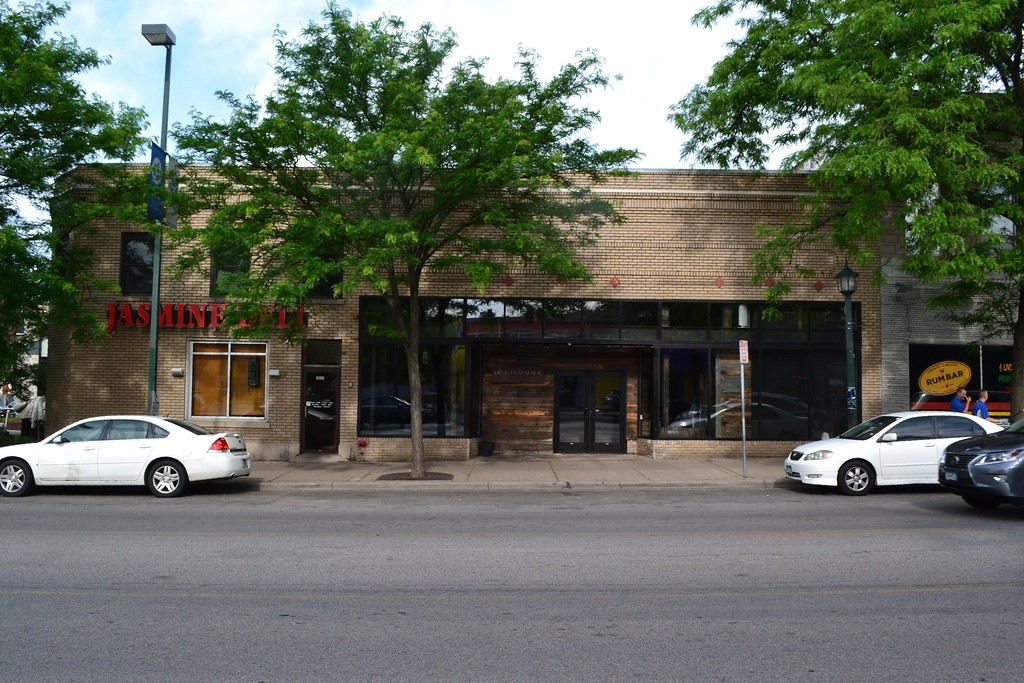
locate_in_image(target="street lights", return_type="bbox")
[139,23,177,415]
[835,257,860,432]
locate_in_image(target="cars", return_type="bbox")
[668,398,809,441]
[784,410,1006,496]
[360,394,434,424]
[0,415,253,498]
[937,415,1024,510]
[673,391,829,428]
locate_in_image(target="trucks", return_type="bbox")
[583,381,686,425]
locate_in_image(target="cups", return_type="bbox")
[1002,419,1008,425]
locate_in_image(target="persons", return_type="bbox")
[950,387,971,413]
[971,390,1002,424]
